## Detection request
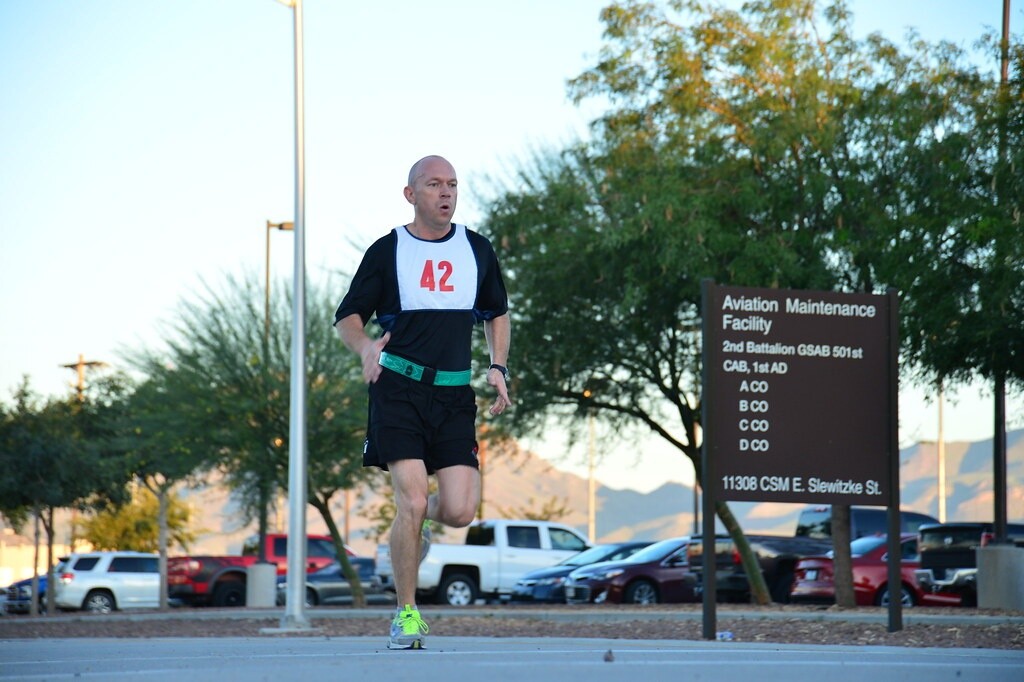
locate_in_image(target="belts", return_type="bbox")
[379,351,471,386]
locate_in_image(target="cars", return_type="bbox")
[0,502,1024,615]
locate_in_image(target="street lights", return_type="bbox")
[253,217,297,564]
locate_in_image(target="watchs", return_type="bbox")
[488,364,509,380]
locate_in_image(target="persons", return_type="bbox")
[332,155,511,650]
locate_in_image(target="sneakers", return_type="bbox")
[419,520,430,561]
[390,606,429,650]
